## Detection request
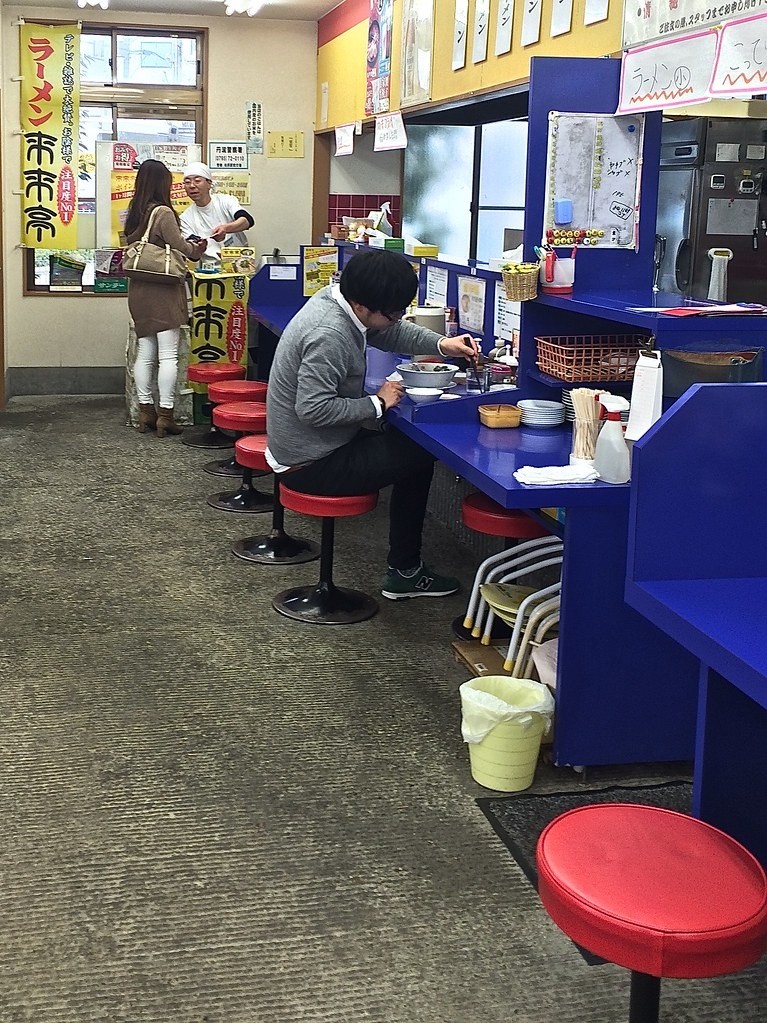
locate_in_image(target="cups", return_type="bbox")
[539,259,574,294]
[466,369,486,396]
[446,323,458,340]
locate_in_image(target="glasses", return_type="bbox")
[380,307,405,322]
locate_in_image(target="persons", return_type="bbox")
[124,160,200,437]
[265,249,479,599]
[178,162,254,268]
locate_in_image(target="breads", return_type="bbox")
[348,219,373,237]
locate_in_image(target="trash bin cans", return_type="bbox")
[460,677,551,792]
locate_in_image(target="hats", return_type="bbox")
[183,162,211,180]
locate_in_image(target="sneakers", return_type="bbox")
[381,559,461,600]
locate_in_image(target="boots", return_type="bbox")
[138,403,158,432]
[156,405,184,438]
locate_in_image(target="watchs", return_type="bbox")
[377,397,385,414]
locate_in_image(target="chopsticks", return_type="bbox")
[464,337,483,392]
[569,389,604,459]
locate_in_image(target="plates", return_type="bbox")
[368,20,380,68]
[398,380,457,389]
[518,388,630,429]
[439,394,462,400]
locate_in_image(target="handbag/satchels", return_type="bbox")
[122,206,188,285]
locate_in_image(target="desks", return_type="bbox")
[246,238,701,783]
[621,383,767,858]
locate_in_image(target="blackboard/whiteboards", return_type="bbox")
[541,109,645,250]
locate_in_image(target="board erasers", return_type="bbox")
[555,199,573,224]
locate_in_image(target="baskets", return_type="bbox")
[534,332,654,382]
[501,262,541,300]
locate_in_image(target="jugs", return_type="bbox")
[402,306,446,337]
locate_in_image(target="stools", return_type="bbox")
[187,362,767,1023]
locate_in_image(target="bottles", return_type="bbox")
[405,305,412,313]
[487,336,518,375]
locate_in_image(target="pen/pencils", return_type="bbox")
[533,244,578,260]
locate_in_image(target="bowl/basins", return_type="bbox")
[490,364,512,382]
[490,384,515,393]
[396,362,459,387]
[406,387,444,406]
[478,404,521,427]
